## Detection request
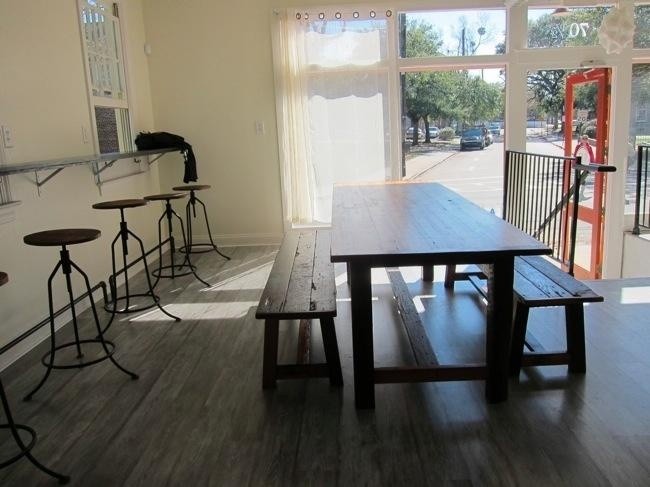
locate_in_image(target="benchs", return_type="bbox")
[444,255,604,378]
[255,228,344,390]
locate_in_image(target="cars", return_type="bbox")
[406,126,424,139]
[461,123,503,150]
[425,126,441,138]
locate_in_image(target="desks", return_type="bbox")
[330,182,552,408]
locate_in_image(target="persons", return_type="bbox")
[573,135,596,202]
